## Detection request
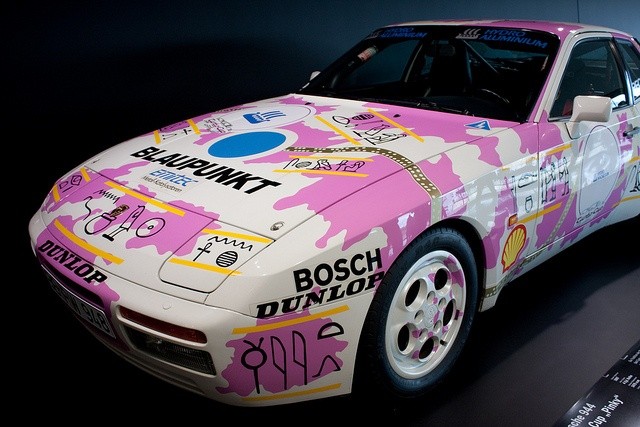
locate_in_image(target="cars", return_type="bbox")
[27,16,640,410]
